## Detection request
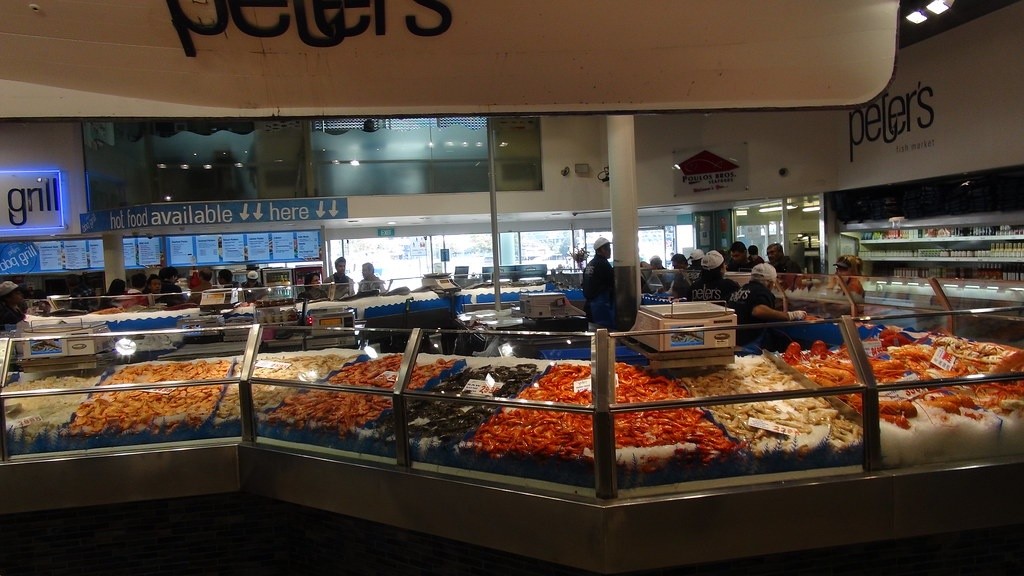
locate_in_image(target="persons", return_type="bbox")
[582,239,614,327]
[0,281,28,331]
[720,241,764,272]
[186,268,212,306]
[767,242,802,274]
[358,263,386,293]
[99,266,185,310]
[218,269,245,304]
[321,257,355,301]
[671,249,705,302]
[65,274,93,312]
[640,254,687,294]
[297,271,328,302]
[682,250,807,325]
[241,270,267,306]
[735,262,777,357]
[822,255,865,318]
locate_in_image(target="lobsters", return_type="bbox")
[782,329,1024,429]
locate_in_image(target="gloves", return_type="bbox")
[787,310,808,322]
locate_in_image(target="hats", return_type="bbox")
[833,255,861,275]
[689,249,704,261]
[247,271,259,280]
[593,237,614,250]
[701,249,725,271]
[0,281,19,297]
[750,262,777,280]
[648,255,660,262]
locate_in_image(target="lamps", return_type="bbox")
[906,1,955,24]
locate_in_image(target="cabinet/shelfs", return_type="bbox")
[838,211,1024,301]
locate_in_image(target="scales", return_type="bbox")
[199,288,239,306]
[520,292,587,319]
[177,312,225,335]
[309,309,356,337]
[21,317,117,360]
[628,304,737,352]
[422,272,462,292]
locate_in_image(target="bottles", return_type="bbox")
[978,263,1024,281]
[991,243,1024,257]
[267,275,289,281]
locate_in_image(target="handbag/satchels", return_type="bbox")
[63,271,102,311]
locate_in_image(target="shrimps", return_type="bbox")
[2,375,100,436]
[219,353,747,468]
[68,359,231,436]
[682,358,863,454]
